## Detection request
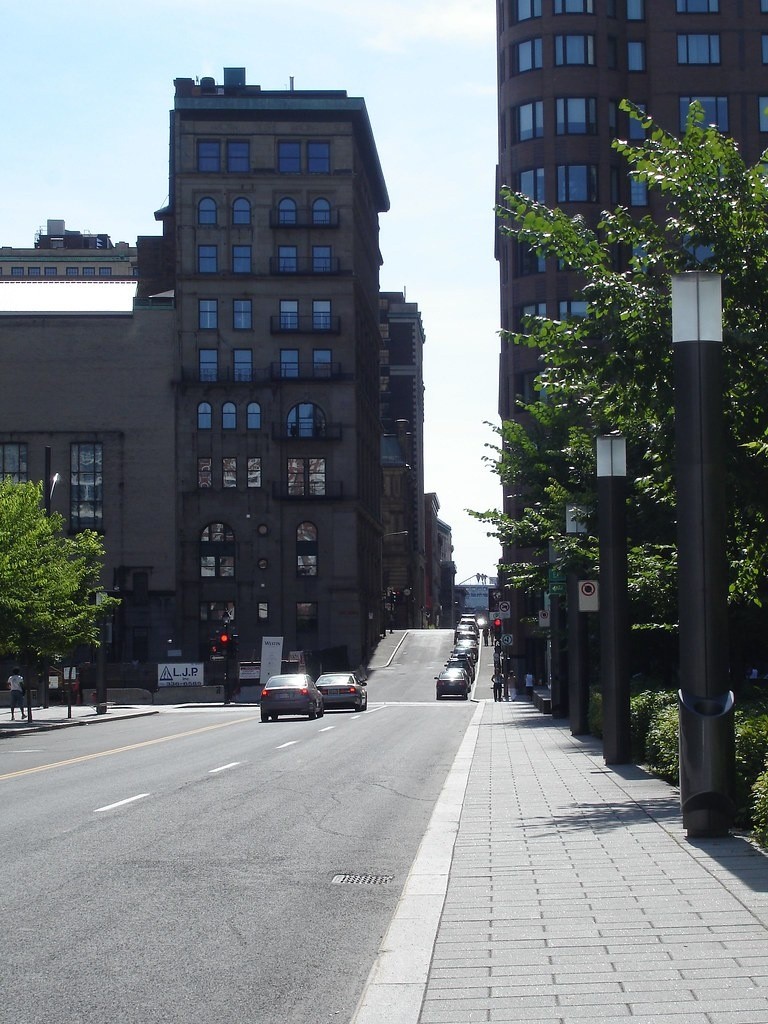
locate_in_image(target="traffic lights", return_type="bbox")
[230,634,239,654]
[221,633,229,645]
[391,591,397,604]
[495,618,501,642]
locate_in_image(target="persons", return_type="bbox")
[491,668,505,702]
[483,626,489,647]
[7,666,27,719]
[507,671,518,702]
[524,670,535,701]
[490,626,496,646]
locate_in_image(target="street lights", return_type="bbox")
[565,502,595,735]
[595,428,638,767]
[670,268,736,838]
[380,530,409,611]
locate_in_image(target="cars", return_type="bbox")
[433,667,469,701]
[260,674,325,722]
[444,613,480,684]
[316,671,369,713]
